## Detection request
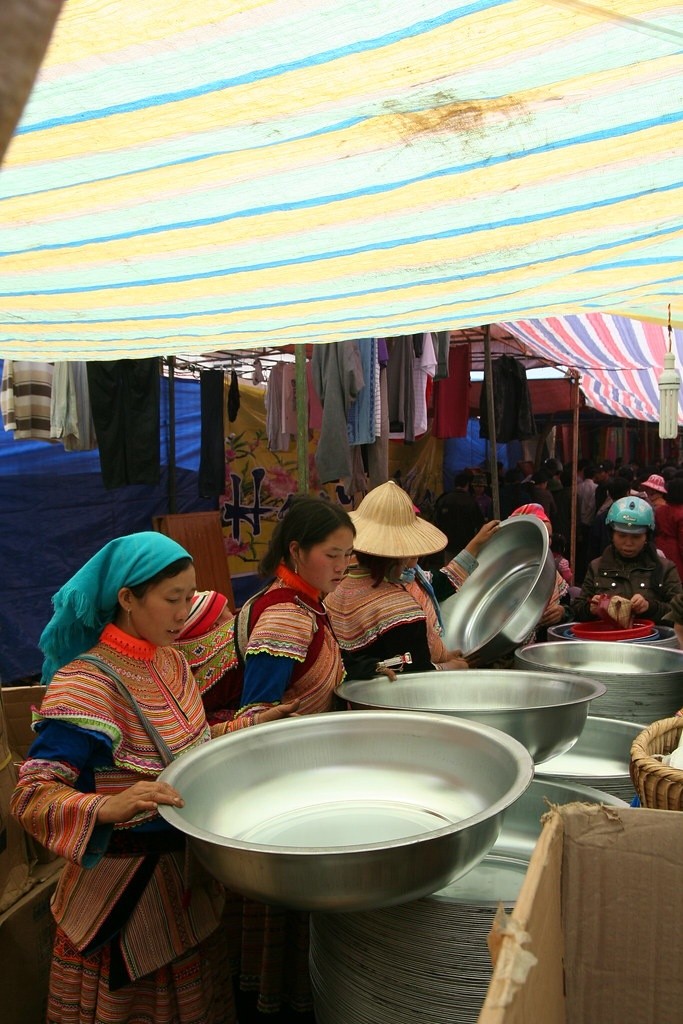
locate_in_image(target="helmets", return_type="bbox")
[606,497,656,532]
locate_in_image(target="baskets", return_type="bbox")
[626,714,683,811]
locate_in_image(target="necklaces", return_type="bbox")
[294,595,328,616]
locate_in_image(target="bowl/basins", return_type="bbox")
[159,514,679,1024]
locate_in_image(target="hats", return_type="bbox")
[642,474,666,493]
[506,504,550,522]
[347,480,448,557]
[176,591,227,644]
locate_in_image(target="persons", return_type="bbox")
[9,532,304,1024]
[400,518,502,663]
[171,589,244,724]
[320,480,471,683]
[230,492,399,1024]
[437,457,683,669]
